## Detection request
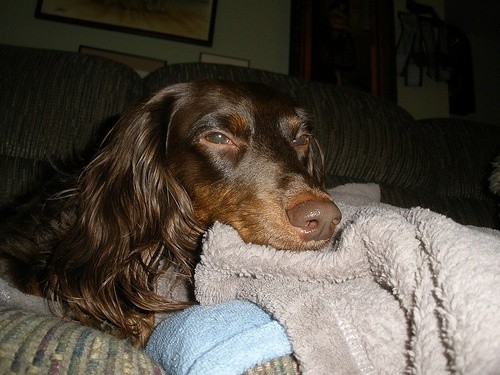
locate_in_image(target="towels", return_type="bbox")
[134,298,292,370]
[190,182,498,371]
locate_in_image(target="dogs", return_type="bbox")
[0,80,342,348]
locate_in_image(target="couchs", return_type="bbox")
[0,42,500,375]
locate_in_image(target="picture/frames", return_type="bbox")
[287,0,398,104]
[200,51,250,68]
[35,0,219,46]
[77,44,168,80]
